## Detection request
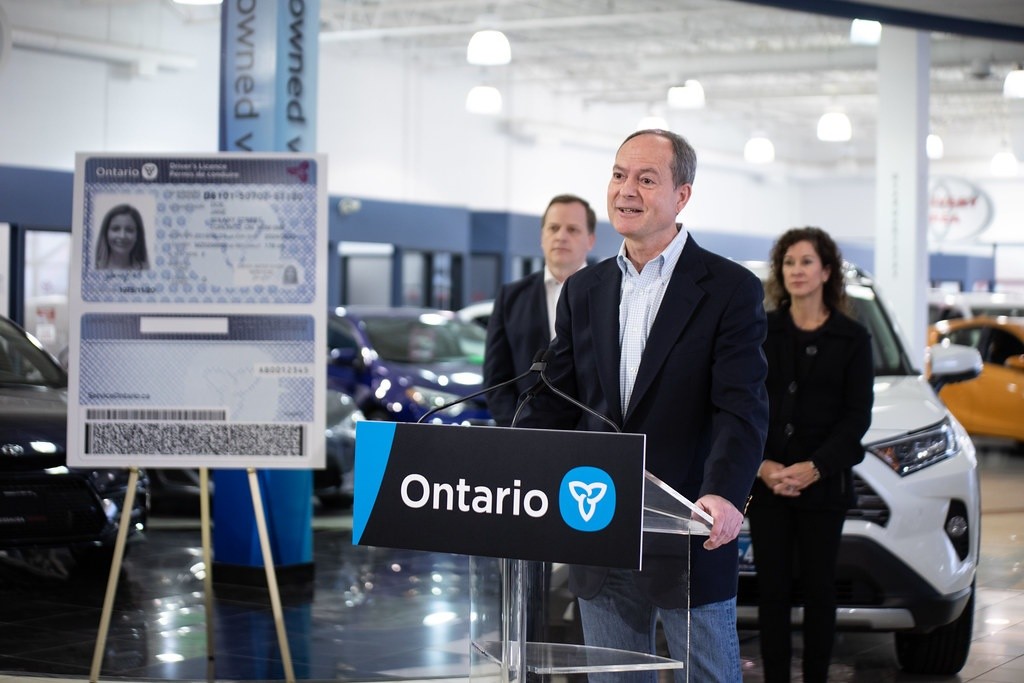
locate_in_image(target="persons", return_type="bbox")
[484,195,597,683]
[750,227,874,683]
[94,204,148,271]
[516,129,769,683]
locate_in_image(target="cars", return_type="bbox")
[326,306,500,428]
[925,318,1024,447]
[928,292,1024,347]
[0,314,151,575]
[28,343,370,508]
[418,302,492,356]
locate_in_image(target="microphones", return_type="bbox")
[540,349,620,433]
[417,349,547,423]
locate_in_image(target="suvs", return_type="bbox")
[703,259,983,672]
